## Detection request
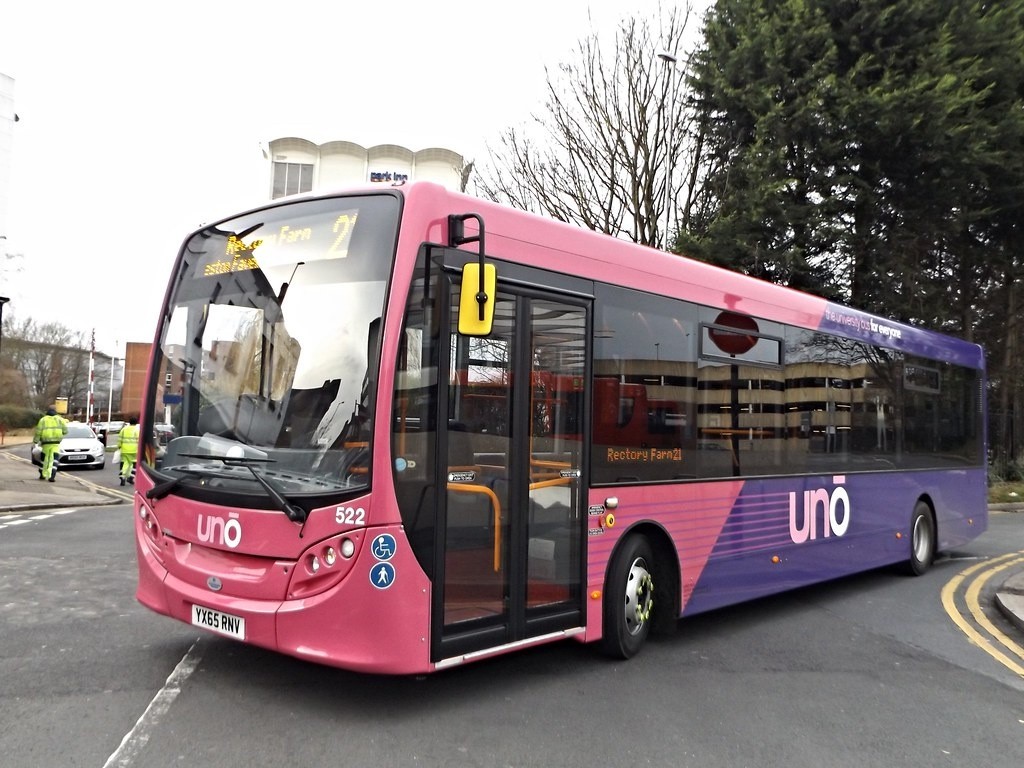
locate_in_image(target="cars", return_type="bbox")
[31,421,106,471]
[93,422,130,433]
[155,424,176,445]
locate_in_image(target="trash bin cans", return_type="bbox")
[100,429,108,447]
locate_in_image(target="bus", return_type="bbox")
[133,180,988,676]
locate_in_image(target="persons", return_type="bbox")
[33,405,68,482]
[117,416,141,486]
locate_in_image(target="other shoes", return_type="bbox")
[48,479,54,482]
[121,480,125,485]
[40,476,45,479]
[127,479,134,484]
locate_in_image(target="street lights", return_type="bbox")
[0,296,10,351]
[288,262,305,286]
[657,52,676,252]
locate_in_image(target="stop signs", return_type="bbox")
[708,312,760,355]
